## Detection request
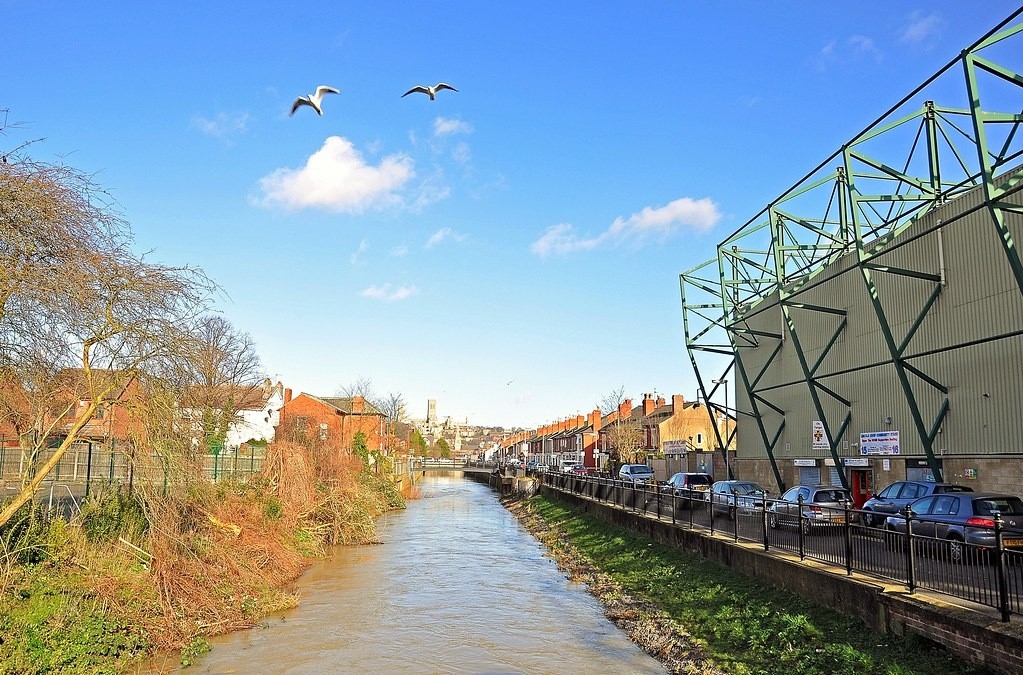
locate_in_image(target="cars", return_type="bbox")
[704,480,774,521]
[618,464,656,488]
[571,464,589,478]
[510,458,549,472]
[659,472,716,509]
[768,483,858,536]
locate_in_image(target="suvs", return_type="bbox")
[883,491,1023,564]
[860,481,976,529]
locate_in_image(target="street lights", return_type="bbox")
[711,379,729,480]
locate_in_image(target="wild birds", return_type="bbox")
[400,82,460,101]
[287,85,343,117]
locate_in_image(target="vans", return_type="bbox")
[558,460,583,474]
